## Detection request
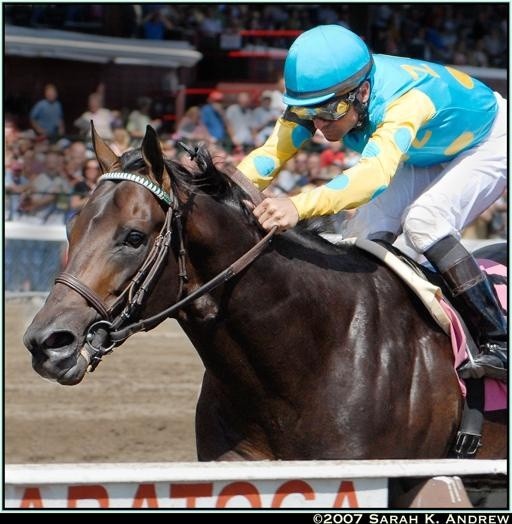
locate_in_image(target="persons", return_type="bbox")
[228,23,509,380]
[5,6,509,222]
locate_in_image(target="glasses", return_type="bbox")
[290,91,357,121]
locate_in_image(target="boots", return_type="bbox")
[451,272,508,380]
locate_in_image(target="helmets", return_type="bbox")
[283,24,376,106]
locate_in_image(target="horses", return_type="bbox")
[21,117,507,508]
